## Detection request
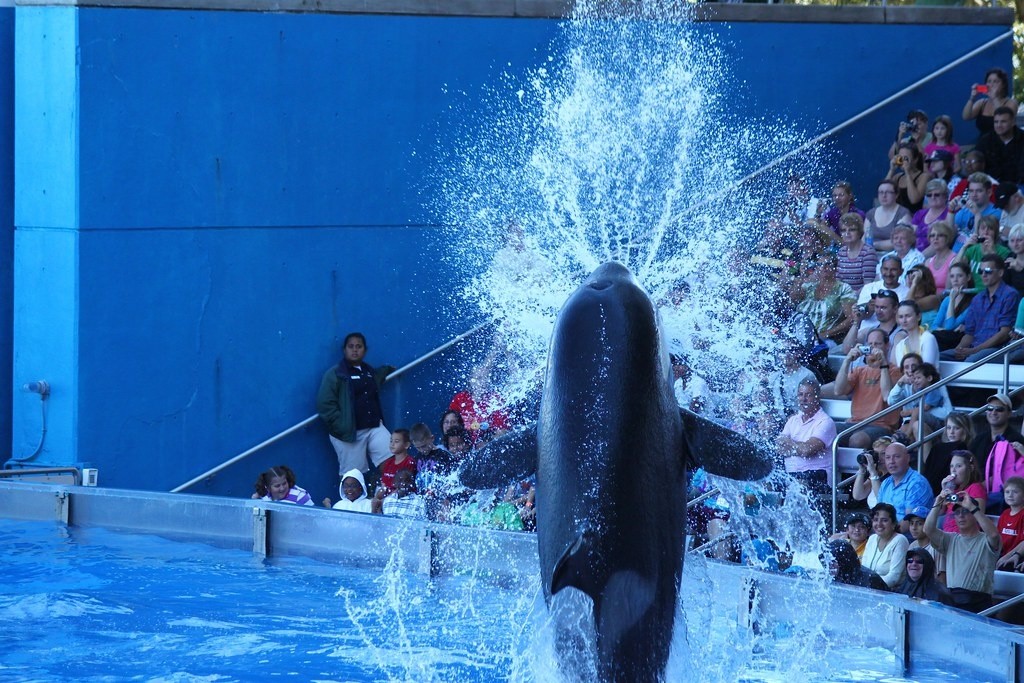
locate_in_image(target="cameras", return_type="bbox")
[944,494,959,503]
[1012,327,1024,337]
[905,121,919,132]
[857,346,872,354]
[958,198,968,206]
[976,236,986,243]
[896,156,909,165]
[857,451,878,464]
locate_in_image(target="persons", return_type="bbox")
[322,365,543,531]
[670,69,1024,616]
[251,465,316,506]
[316,333,395,476]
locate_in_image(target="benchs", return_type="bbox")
[815,446,866,502]
[828,353,1024,412]
[818,398,854,427]
[992,570,1024,601]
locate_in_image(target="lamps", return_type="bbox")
[22,380,50,395]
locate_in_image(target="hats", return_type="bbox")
[952,496,979,511]
[923,149,953,163]
[846,513,872,531]
[994,182,1019,209]
[903,506,930,520]
[987,393,1012,411]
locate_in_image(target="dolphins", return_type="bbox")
[457,259,773,683]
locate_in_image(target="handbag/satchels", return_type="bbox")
[788,313,835,385]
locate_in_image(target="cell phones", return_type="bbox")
[976,85,989,93]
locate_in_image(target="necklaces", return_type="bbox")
[870,534,892,571]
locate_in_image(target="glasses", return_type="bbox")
[926,193,941,198]
[985,405,1009,412]
[878,289,894,297]
[978,268,1001,274]
[953,509,970,516]
[907,558,924,564]
[877,191,896,196]
[963,160,982,165]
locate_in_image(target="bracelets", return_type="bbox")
[869,476,879,480]
[857,471,865,475]
[526,502,532,506]
[1012,551,1021,561]
[880,365,889,369]
[970,507,981,515]
[814,214,821,218]
